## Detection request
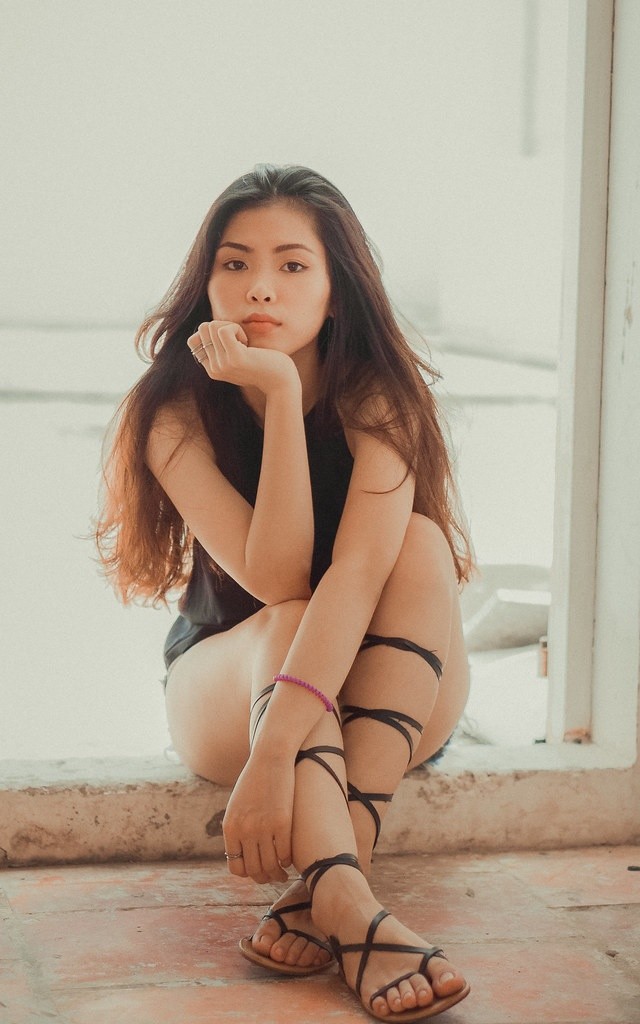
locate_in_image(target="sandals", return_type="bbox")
[326,909,473,1022]
[239,891,339,977]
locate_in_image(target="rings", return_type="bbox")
[190,343,207,363]
[202,343,212,349]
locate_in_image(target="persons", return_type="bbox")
[94,165,482,1017]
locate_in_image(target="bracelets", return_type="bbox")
[274,673,335,714]
